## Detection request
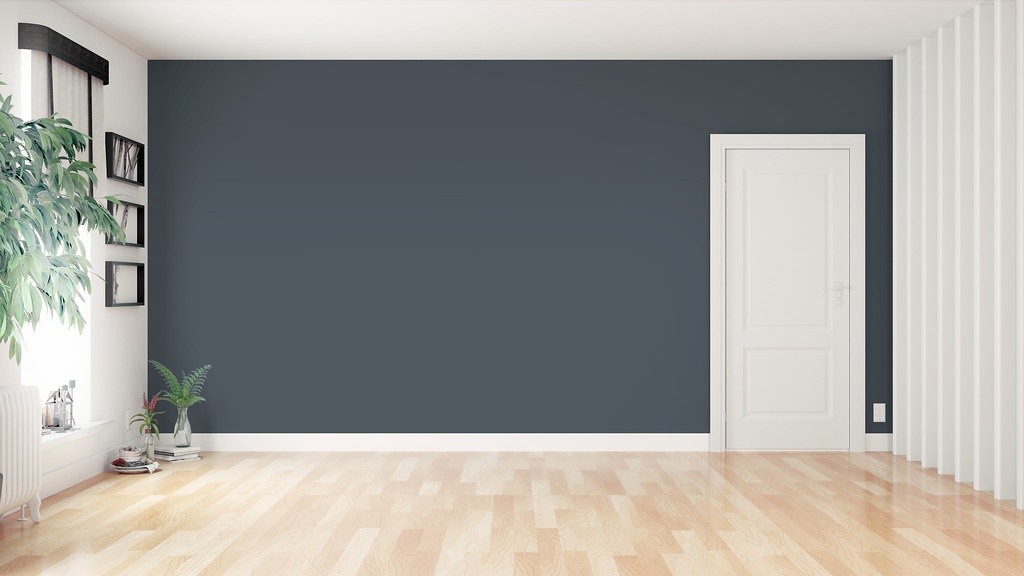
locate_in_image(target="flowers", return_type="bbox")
[129,392,167,440]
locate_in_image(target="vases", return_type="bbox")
[145,428,154,461]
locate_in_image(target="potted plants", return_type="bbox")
[149,358,213,449]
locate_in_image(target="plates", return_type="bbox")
[111,464,148,473]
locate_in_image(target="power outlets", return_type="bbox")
[873,403,886,422]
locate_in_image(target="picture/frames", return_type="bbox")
[105,132,145,187]
[106,198,144,248]
[105,261,145,306]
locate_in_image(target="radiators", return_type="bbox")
[0,385,42,524]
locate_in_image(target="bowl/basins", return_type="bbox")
[119,446,141,461]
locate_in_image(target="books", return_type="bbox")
[154,446,203,463]
